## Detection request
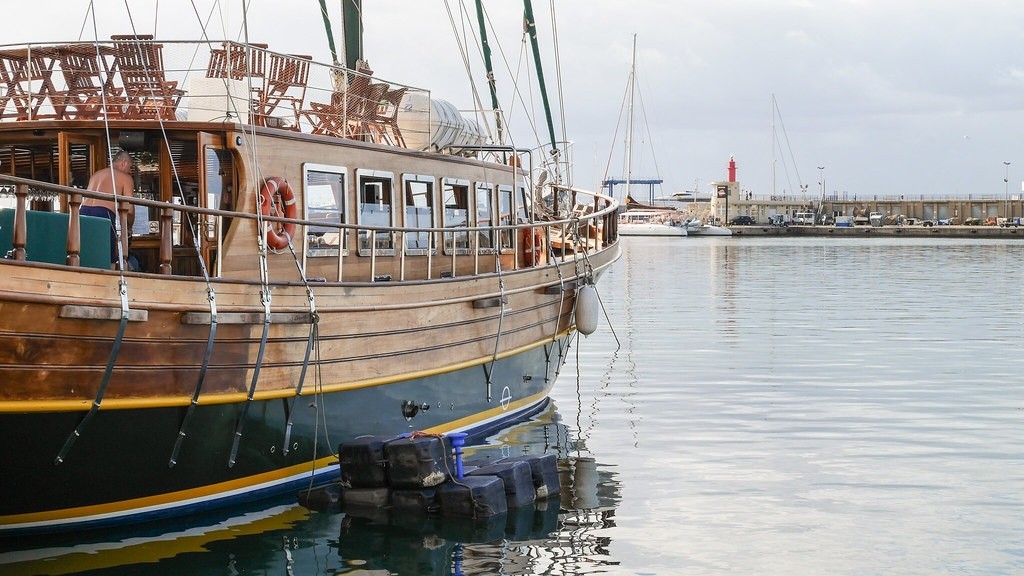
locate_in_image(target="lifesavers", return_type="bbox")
[256,177,298,248]
[524,228,544,266]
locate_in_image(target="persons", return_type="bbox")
[80,151,135,270]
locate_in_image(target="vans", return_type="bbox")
[792,213,816,225]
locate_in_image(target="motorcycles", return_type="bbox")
[768,214,790,227]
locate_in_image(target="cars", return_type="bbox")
[730,215,758,225]
[869,211,996,227]
[817,214,834,225]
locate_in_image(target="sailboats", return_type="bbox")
[604,32,690,238]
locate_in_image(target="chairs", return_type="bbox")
[248,53,312,131]
[0,34,189,122]
[309,67,408,149]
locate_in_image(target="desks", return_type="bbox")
[0,44,124,122]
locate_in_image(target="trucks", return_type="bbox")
[999,217,1020,227]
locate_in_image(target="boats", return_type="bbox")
[0,0,635,550]
[678,219,734,238]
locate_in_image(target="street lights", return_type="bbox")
[800,184,808,226]
[772,160,777,195]
[1003,161,1010,217]
[817,164,825,200]
